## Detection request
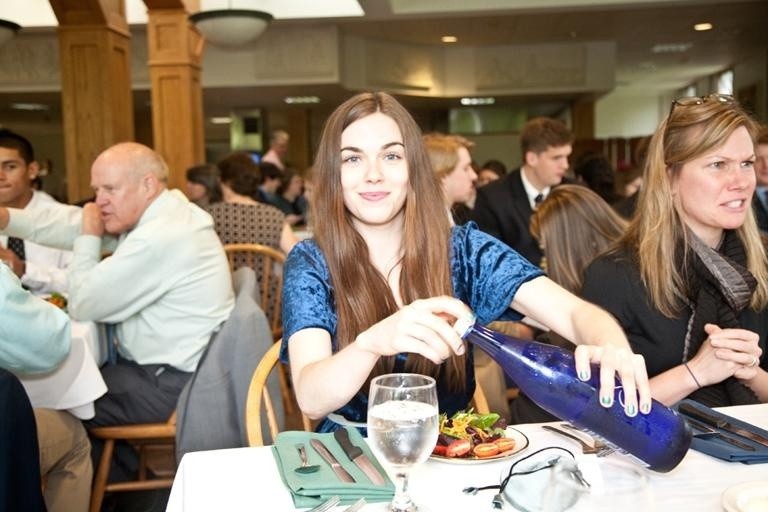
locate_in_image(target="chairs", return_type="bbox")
[223,243,285,340]
[244,336,491,447]
[89,268,274,512]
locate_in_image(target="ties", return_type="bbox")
[535,194,544,205]
[7,236,30,291]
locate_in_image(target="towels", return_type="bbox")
[272,423,396,509]
[669,399,768,464]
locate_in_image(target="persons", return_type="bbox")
[1,143,237,511]
[0,368,95,511]
[0,93,768,436]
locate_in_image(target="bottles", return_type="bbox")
[453,312,694,476]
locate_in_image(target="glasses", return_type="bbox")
[668,92,736,121]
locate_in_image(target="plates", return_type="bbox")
[428,411,531,466]
[38,291,77,313]
[721,479,768,512]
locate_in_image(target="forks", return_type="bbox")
[540,423,614,458]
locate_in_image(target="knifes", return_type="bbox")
[678,401,768,457]
[295,427,387,512]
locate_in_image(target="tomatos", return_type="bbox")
[495,438,516,451]
[473,444,497,456]
[447,439,470,457]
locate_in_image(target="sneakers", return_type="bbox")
[105,465,162,512]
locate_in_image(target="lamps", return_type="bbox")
[0,20,22,46]
[188,0,273,47]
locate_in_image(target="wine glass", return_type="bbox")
[367,371,436,512]
